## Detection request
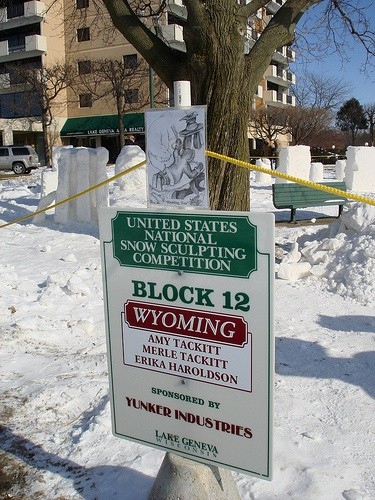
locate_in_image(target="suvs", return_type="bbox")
[0,145,39,175]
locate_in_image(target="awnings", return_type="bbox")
[60,112,145,137]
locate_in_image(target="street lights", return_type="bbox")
[141,12,163,108]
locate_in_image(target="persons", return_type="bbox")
[260,139,282,170]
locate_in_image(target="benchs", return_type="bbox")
[272,182,352,225]
